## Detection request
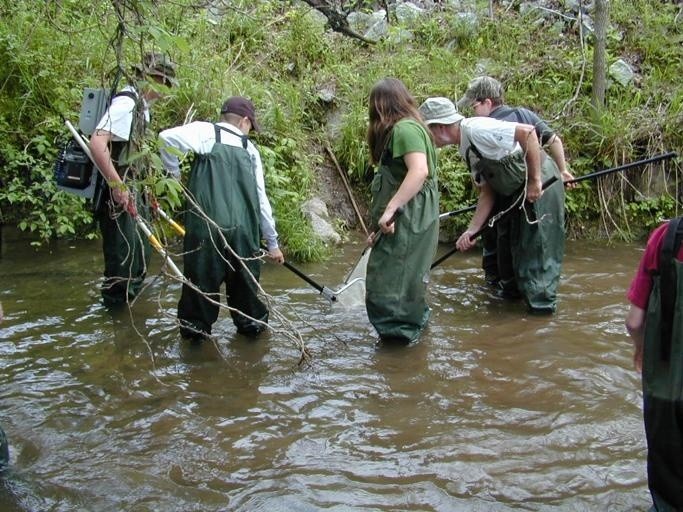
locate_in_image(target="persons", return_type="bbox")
[157,98,284,346]
[89,52,176,307]
[417,96,566,314]
[625,215,683,512]
[365,79,440,345]
[456,77,577,189]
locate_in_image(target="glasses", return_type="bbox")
[470,102,481,109]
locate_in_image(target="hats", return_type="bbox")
[132,54,180,88]
[456,76,501,109]
[220,97,259,130]
[416,96,464,127]
